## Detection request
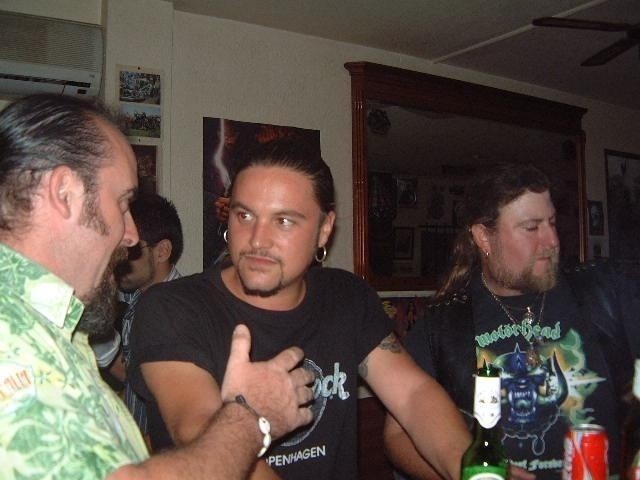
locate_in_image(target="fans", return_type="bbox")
[532,15,640,67]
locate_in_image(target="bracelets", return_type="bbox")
[220,393,273,459]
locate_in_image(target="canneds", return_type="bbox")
[562,423,609,480]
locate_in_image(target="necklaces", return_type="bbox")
[479,275,548,368]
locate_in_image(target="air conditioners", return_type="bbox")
[0,10,104,102]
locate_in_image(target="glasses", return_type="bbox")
[128,241,146,262]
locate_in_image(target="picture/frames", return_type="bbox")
[114,64,166,206]
[392,226,414,260]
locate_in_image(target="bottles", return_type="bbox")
[460,368,509,480]
[620,356,640,478]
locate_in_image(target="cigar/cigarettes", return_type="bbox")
[219,202,226,209]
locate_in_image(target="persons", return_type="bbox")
[0,96,318,480]
[203,126,297,249]
[91,327,129,383]
[81,189,185,455]
[379,161,640,480]
[123,140,475,480]
